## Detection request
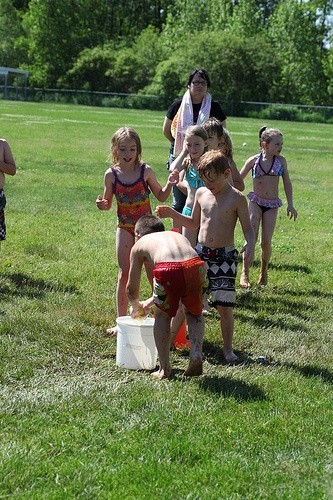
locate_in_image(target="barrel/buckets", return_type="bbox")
[115,316,158,370]
[153,309,186,344]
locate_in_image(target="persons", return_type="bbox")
[126,212,208,379]
[154,150,255,364]
[96,126,180,337]
[0,137,16,241]
[163,68,245,255]
[239,126,299,288]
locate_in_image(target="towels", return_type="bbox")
[171,89,211,158]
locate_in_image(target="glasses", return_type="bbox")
[192,80,207,86]
